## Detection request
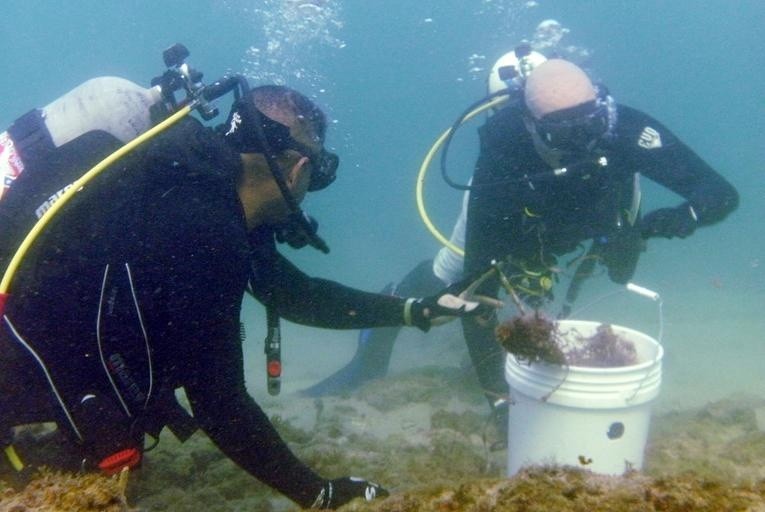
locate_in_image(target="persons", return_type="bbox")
[0,84,504,511]
[289,57,742,448]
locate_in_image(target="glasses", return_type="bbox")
[288,138,340,193]
[525,100,611,153]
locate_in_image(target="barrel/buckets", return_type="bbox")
[504,283,664,475]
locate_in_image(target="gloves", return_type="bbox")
[411,273,498,332]
[638,193,714,242]
[489,397,509,451]
[309,474,391,512]
